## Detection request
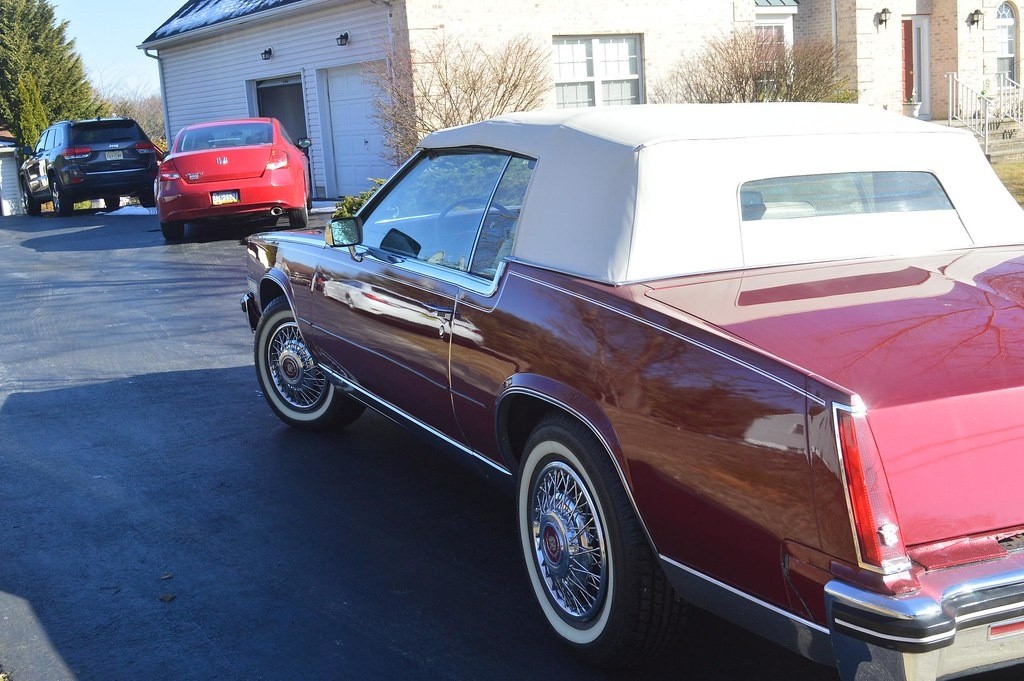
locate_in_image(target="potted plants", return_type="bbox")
[902,87,922,119]
[977,78,996,118]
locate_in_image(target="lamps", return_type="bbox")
[336,32,348,46]
[971,9,984,28]
[261,48,272,60]
[879,8,893,29]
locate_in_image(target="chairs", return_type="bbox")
[192,132,265,151]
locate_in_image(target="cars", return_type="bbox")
[154,117,312,239]
[237,102,1024,681]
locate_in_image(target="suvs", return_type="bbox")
[18,115,158,217]
